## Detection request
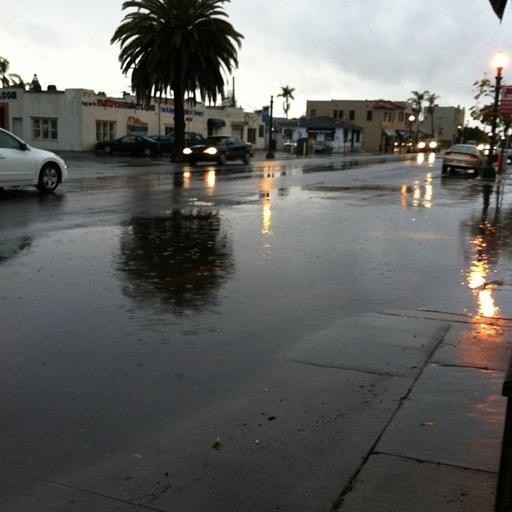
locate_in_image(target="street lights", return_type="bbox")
[485,45,512,179]
[456,124,464,144]
[407,113,416,155]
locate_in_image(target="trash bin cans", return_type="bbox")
[297,138,308,155]
[479,148,498,181]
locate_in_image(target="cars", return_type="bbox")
[415,138,512,177]
[0,126,66,195]
[94,115,254,165]
[282,136,336,156]
